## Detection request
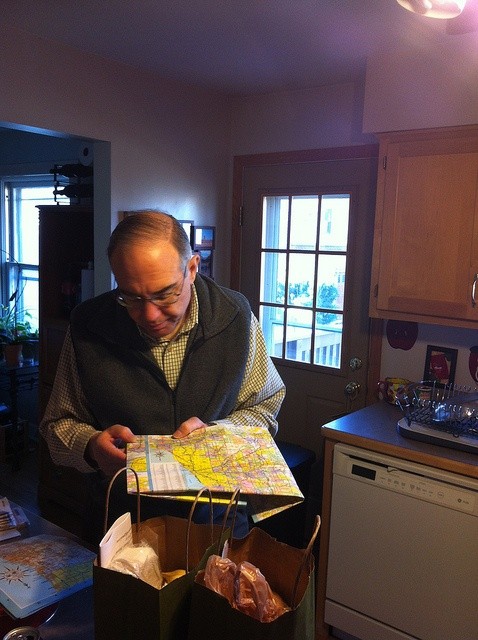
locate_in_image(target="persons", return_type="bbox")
[39,211,288,547]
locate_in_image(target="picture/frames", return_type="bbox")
[193,250,214,280]
[423,344,458,391]
[118,210,138,222]
[192,226,216,250]
[178,220,194,245]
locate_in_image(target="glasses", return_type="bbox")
[113,258,190,309]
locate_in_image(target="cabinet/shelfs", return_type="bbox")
[368,124,477,332]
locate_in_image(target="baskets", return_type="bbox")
[394,380,478,439]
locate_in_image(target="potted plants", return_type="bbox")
[0,288,37,366]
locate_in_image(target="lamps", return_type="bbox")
[396,0,468,19]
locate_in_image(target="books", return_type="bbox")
[0,497,30,542]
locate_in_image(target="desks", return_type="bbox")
[0,360,39,472]
[0,497,99,640]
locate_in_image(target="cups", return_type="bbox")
[3,626,40,640]
[377,377,411,408]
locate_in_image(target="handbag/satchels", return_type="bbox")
[187,489,322,640]
[93,465,233,640]
